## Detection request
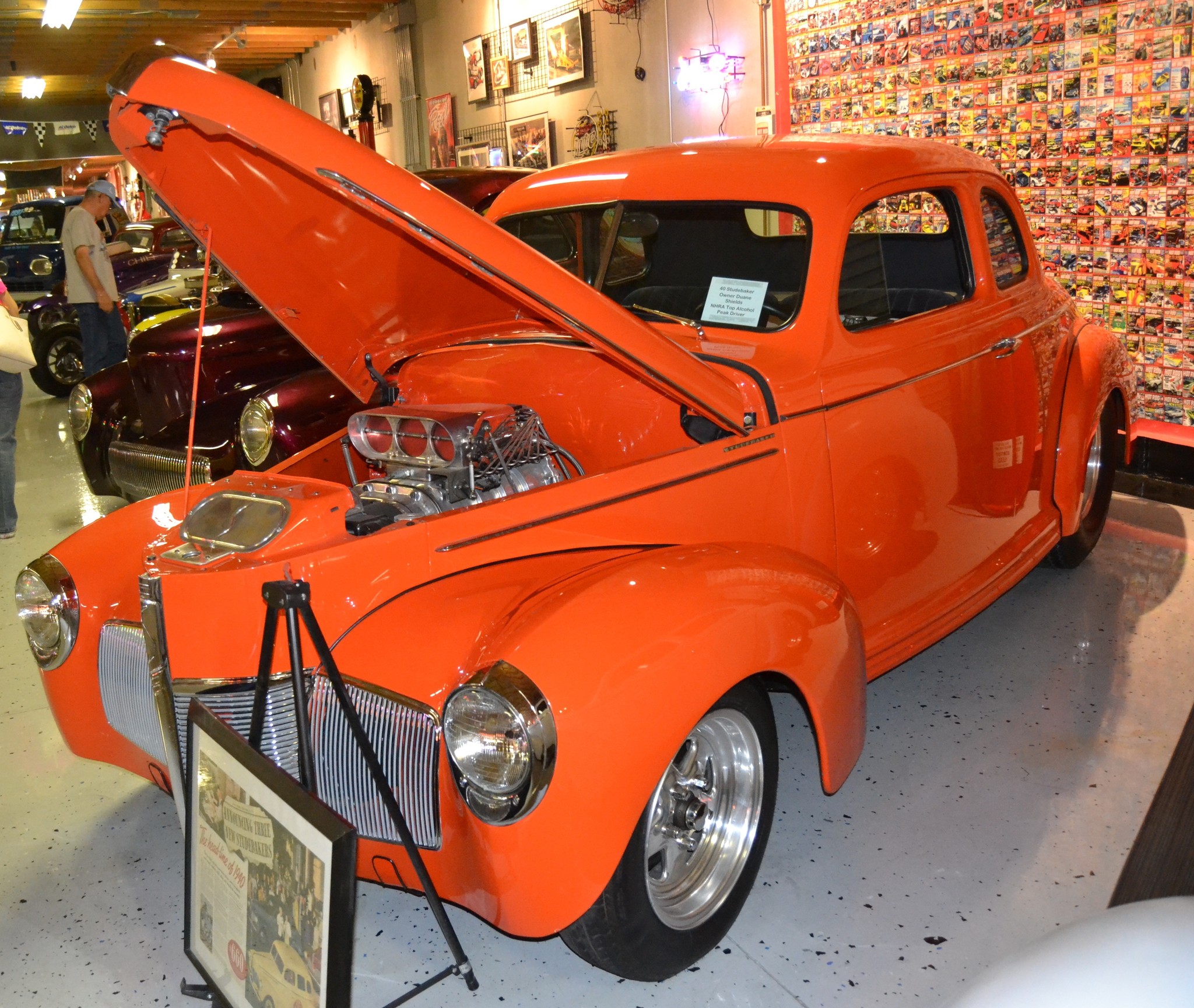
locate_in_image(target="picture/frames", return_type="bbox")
[318,90,343,133]
[542,8,587,88]
[503,111,553,169]
[456,140,490,168]
[489,55,510,92]
[460,37,487,106]
[507,16,534,65]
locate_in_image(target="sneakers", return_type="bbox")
[0,526,17,539]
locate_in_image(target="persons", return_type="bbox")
[0,276,26,539]
[60,180,129,380]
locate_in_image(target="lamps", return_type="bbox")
[676,51,744,99]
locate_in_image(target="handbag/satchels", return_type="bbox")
[0,305,38,374]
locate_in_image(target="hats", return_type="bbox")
[87,180,123,209]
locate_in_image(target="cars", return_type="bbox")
[0,46,1141,981]
